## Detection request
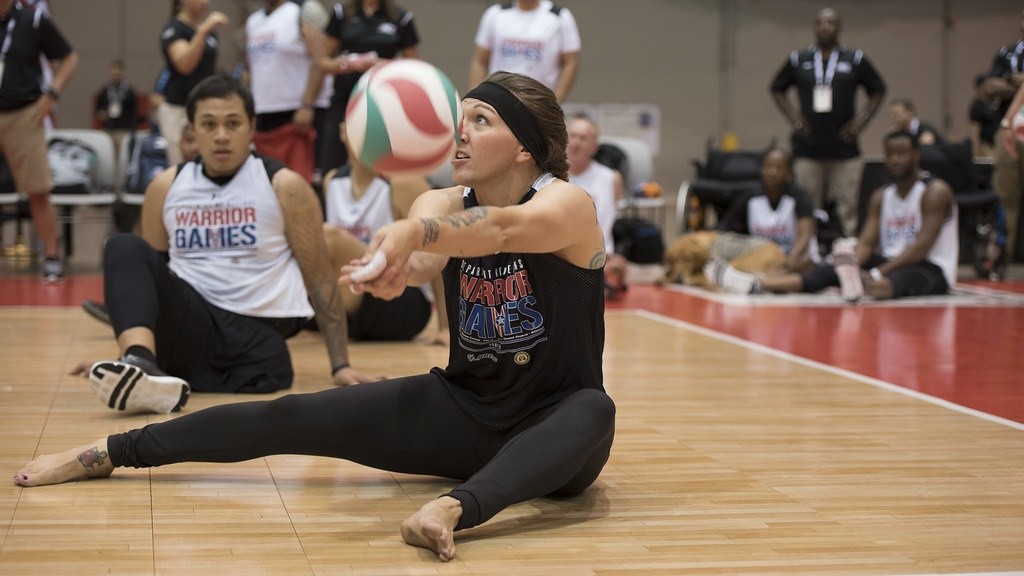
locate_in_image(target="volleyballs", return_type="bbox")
[344,55,464,176]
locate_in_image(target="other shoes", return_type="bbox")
[704,260,761,294]
[84,301,113,325]
[90,353,191,414]
[833,235,865,304]
[43,257,68,284]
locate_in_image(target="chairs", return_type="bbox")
[0,129,148,256]
[563,101,666,229]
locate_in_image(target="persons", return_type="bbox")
[90,75,388,412]
[1,0,76,283]
[969,37,1024,278]
[302,1,420,170]
[837,129,959,302]
[728,145,827,294]
[892,95,951,158]
[769,8,887,233]
[14,70,618,563]
[94,60,139,130]
[471,0,580,104]
[246,0,324,184]
[149,0,228,170]
[564,112,626,288]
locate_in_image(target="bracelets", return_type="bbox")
[871,269,881,279]
[42,85,59,102]
[1001,120,1010,126]
[331,363,349,373]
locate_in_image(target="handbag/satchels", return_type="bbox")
[614,205,665,265]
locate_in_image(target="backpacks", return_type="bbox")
[126,135,169,192]
[44,136,98,193]
